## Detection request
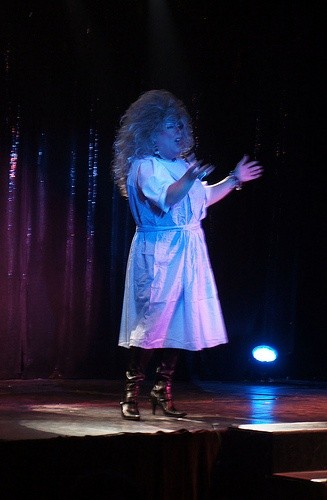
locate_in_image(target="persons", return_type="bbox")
[110,89,264,423]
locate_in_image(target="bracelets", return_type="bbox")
[229,171,242,190]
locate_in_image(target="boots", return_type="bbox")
[121,370,144,419]
[150,368,188,417]
